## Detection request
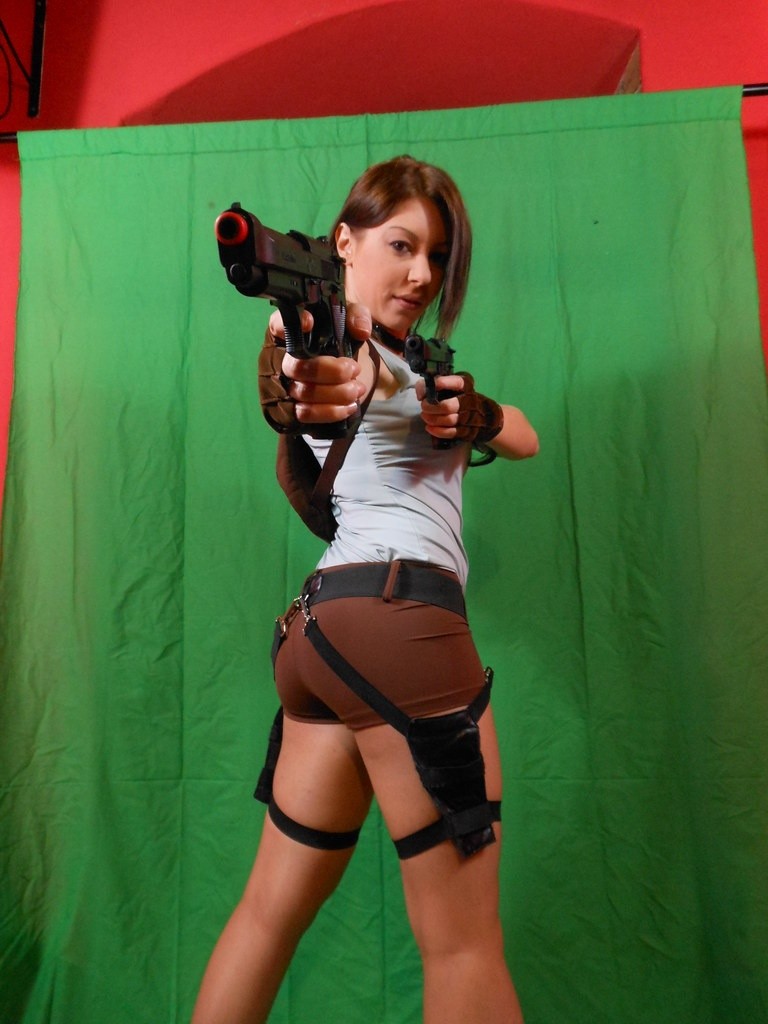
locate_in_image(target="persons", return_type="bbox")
[192,156,539,1024]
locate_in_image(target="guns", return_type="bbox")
[210,199,366,442]
[401,333,462,451]
